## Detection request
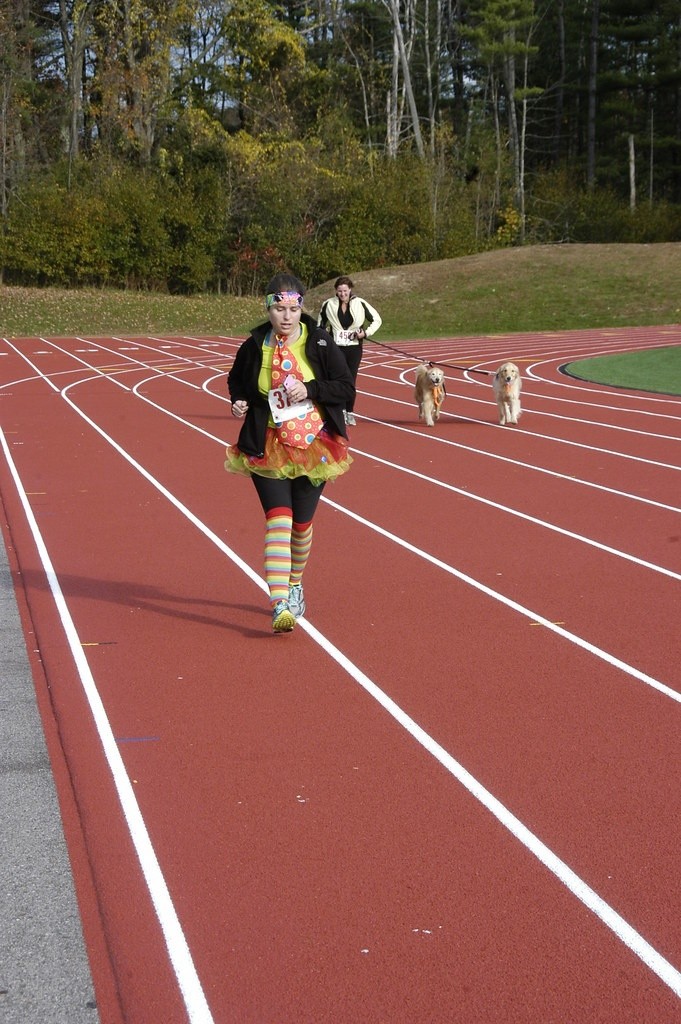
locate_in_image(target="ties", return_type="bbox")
[273,336,326,448]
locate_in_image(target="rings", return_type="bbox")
[295,393,298,400]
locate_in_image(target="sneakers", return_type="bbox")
[272,603,296,633]
[347,413,356,426]
[288,585,304,619]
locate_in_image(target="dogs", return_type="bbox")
[492,362,524,426]
[414,364,447,427]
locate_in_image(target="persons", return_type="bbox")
[316,277,382,426]
[223,274,356,633]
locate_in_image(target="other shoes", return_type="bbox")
[341,409,347,426]
